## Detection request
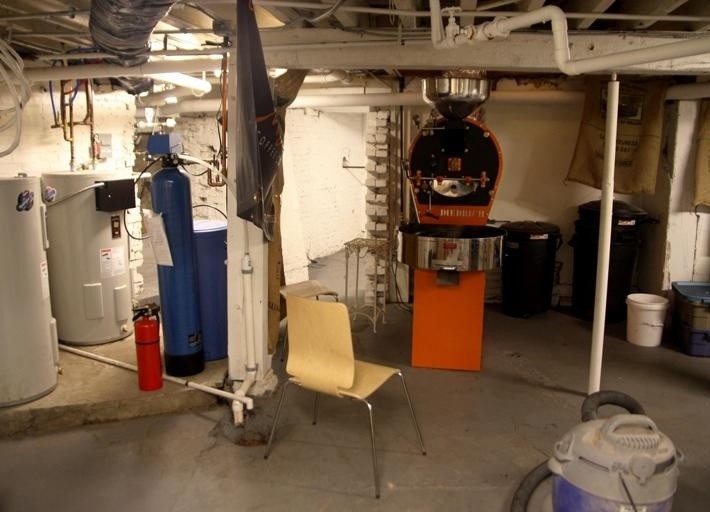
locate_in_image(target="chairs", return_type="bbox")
[280,279,339,361]
[264,292,427,499]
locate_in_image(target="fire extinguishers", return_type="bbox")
[131,304,164,390]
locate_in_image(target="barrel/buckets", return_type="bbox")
[625,291,669,348]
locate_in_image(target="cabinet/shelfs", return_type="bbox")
[344,238,391,334]
